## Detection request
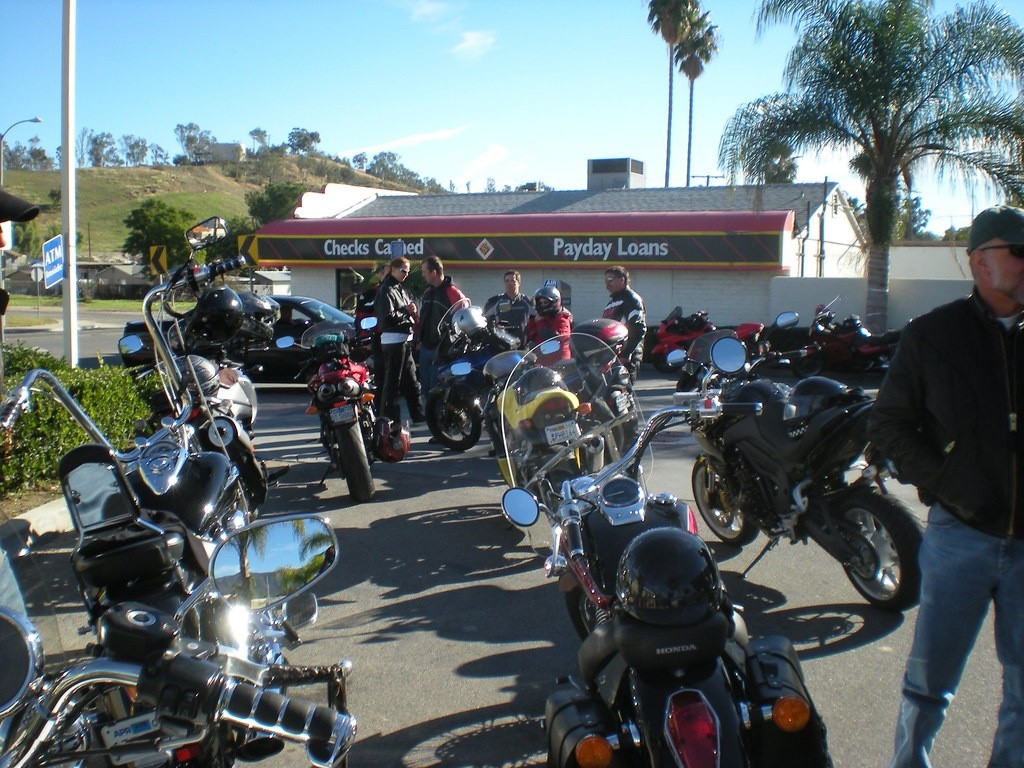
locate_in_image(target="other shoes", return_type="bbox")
[412,413,428,424]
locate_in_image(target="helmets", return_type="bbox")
[514,368,569,405]
[843,314,861,330]
[175,355,221,398]
[786,377,846,438]
[186,284,244,343]
[614,528,721,627]
[453,307,487,334]
[374,417,411,463]
[534,286,562,317]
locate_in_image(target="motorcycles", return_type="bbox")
[665,311,927,614]
[422,297,652,516]
[0,215,358,768]
[649,306,904,381]
[498,330,834,768]
[274,316,376,503]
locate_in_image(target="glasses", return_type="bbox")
[608,275,618,283]
[399,268,411,274]
[982,243,1024,257]
[538,301,549,304]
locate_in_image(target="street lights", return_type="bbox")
[0,117,42,187]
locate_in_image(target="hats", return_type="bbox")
[966,204,1023,256]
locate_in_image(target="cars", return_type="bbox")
[121,295,383,400]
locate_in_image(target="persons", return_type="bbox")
[354,264,426,424]
[866,204,1024,768]
[603,266,648,382]
[417,256,468,443]
[377,257,417,427]
[524,286,572,366]
[484,271,536,348]
